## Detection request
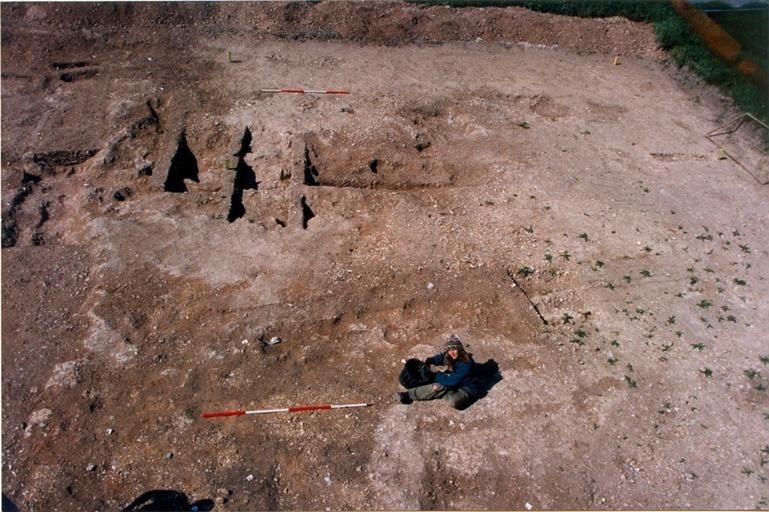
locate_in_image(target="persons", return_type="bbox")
[394,335,487,410]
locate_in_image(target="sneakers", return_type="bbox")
[395,391,409,402]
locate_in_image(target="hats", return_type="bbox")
[444,335,462,350]
